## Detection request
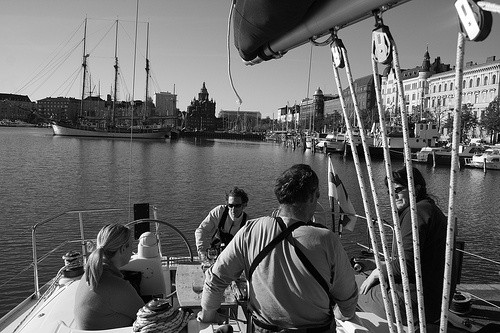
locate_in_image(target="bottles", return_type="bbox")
[220,243,225,253]
[354,262,365,272]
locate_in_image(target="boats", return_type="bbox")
[266,100,500,170]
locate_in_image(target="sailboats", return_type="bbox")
[50,17,172,139]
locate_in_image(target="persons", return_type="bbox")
[197,163,358,333]
[194,187,249,265]
[360,166,456,333]
[73,223,146,330]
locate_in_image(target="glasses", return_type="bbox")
[228,203,243,209]
[387,186,407,194]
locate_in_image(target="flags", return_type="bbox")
[327,157,357,231]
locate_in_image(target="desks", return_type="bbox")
[175,264,238,322]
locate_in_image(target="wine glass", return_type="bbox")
[198,250,207,273]
[192,278,204,300]
[208,248,218,263]
